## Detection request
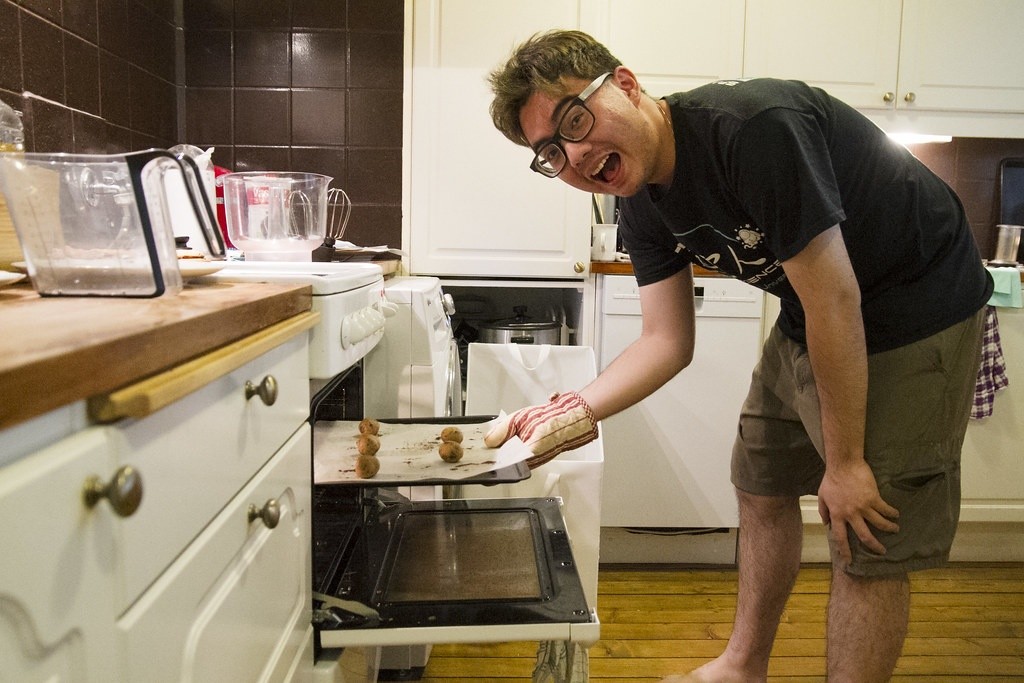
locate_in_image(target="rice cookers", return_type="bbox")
[477,304,561,344]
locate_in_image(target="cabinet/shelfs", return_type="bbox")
[0,312,315,683]
[402,0,603,280]
[742,0,1024,138]
[579,0,745,101]
[764,281,1024,563]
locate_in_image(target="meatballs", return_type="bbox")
[356,456,380,477]
[439,441,463,462]
[357,435,380,456]
[359,418,380,435]
[442,427,463,443]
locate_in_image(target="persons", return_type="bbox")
[484,30,994,683]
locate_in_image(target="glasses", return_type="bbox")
[529,70,614,178]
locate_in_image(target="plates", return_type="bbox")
[12,259,225,279]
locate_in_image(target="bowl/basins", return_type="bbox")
[218,169,335,264]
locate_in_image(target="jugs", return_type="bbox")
[0,149,226,302]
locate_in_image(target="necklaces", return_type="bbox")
[657,102,672,131]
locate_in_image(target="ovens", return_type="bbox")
[310,357,603,655]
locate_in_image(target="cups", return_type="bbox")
[592,223,619,262]
[994,224,1024,262]
